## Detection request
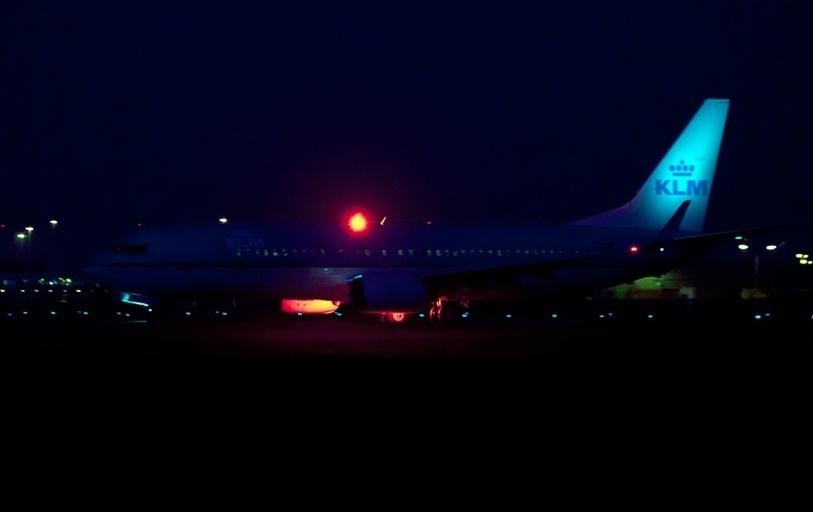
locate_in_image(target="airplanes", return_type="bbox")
[77,92,764,342]
